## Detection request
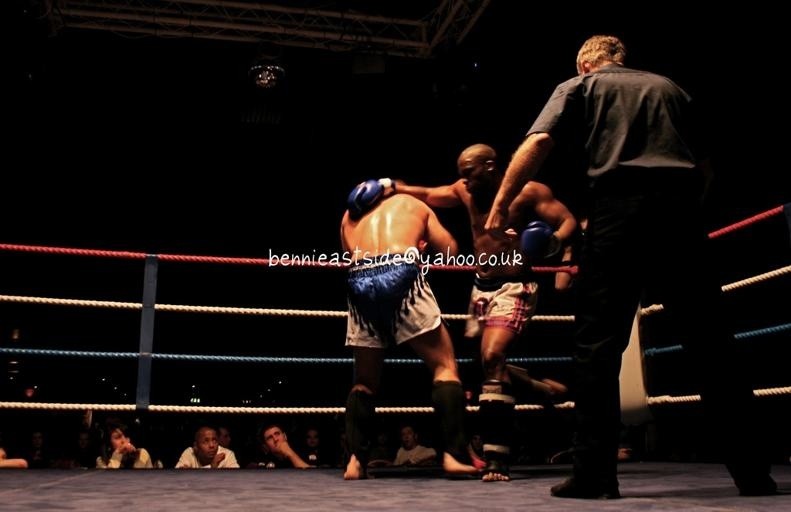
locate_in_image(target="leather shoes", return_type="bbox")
[739,475,777,496]
[551,475,620,499]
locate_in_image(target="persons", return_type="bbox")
[1,35,783,496]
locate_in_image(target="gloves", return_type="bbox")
[346,178,396,217]
[520,220,559,260]
